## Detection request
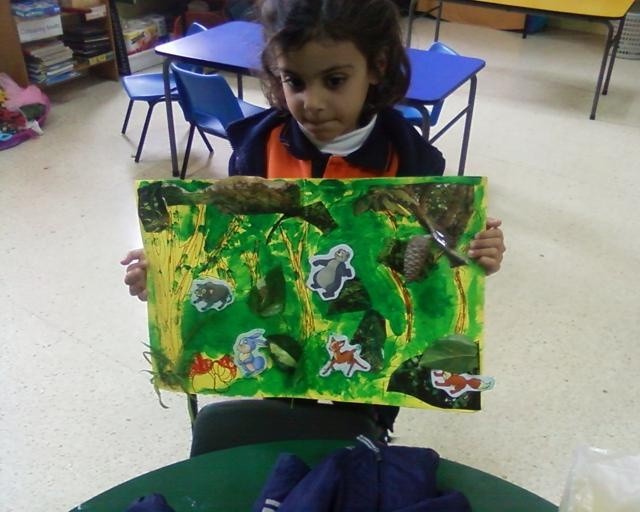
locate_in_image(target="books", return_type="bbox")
[11,0,115,88]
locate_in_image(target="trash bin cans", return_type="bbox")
[608,12,640,60]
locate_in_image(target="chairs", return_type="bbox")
[119,20,269,176]
[393,41,461,140]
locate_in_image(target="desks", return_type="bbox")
[154,18,485,177]
[406,1,635,120]
[68,438,561,512]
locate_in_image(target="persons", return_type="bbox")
[120,0,504,459]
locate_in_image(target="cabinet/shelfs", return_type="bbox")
[0,0,120,99]
